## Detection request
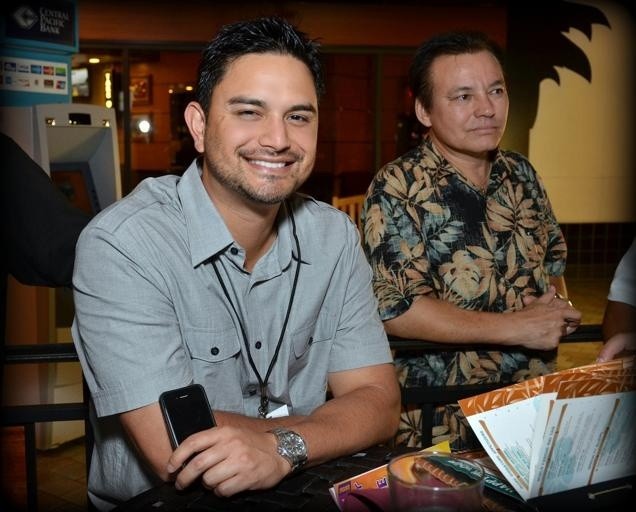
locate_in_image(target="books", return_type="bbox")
[457,353,636,501]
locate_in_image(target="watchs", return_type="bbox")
[265,426,308,476]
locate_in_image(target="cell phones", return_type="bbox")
[160,385,217,471]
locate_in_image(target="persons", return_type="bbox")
[69,16,403,512]
[359,27,583,385]
[594,235,636,365]
[0,129,92,289]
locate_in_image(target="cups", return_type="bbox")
[386,451,484,511]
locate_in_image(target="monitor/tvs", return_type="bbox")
[49,159,101,219]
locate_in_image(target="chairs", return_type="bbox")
[332,191,369,236]
